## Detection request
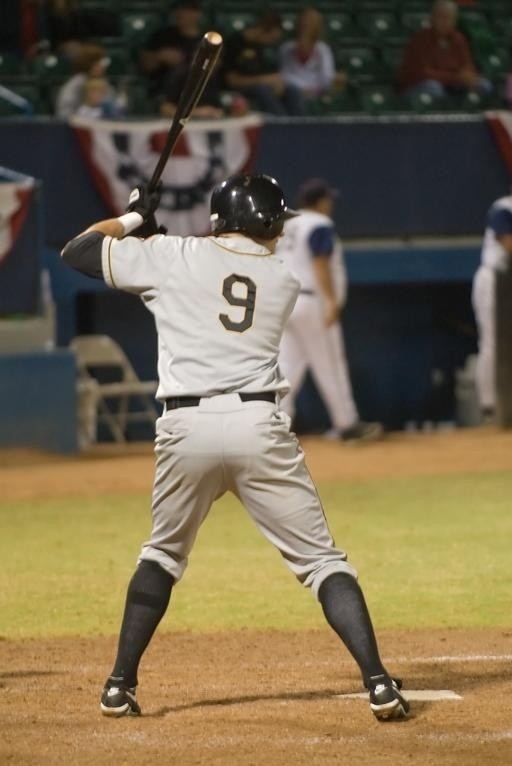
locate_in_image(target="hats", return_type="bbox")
[302,176,344,200]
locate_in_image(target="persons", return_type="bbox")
[273,177,385,440]
[59,171,409,722]
[140,1,235,120]
[36,12,130,120]
[220,3,310,118]
[278,7,345,116]
[465,195,511,427]
[399,0,481,111]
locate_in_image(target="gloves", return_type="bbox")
[129,215,168,239]
[125,175,163,223]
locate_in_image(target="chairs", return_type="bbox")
[69,334,162,447]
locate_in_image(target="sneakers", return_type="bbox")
[368,674,409,720]
[339,419,381,442]
[99,676,141,717]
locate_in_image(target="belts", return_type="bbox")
[165,390,275,410]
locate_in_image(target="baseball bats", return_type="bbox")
[142,32,222,197]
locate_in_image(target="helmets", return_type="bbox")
[208,172,301,241]
